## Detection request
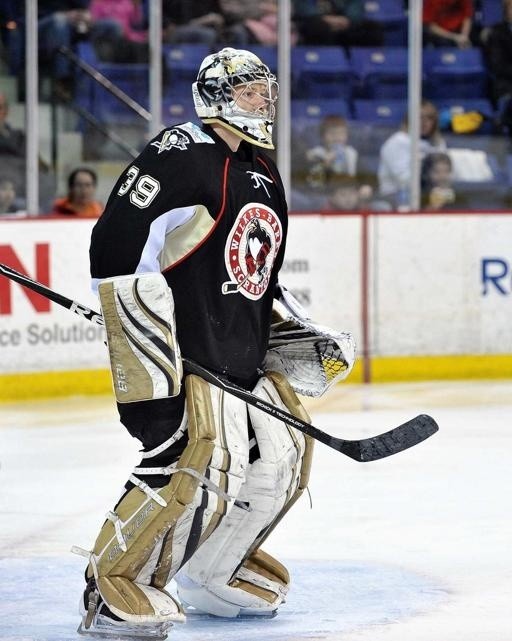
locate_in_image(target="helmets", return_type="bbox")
[190,47,277,148]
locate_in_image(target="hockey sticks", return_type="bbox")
[0,263,439,464]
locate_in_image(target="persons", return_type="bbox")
[307,114,360,186]
[0,176,27,213]
[326,176,357,211]
[0,0,383,106]
[375,101,448,209]
[2,93,27,157]
[417,0,477,50]
[420,152,469,211]
[480,1,512,113]
[81,46,355,628]
[55,168,106,218]
